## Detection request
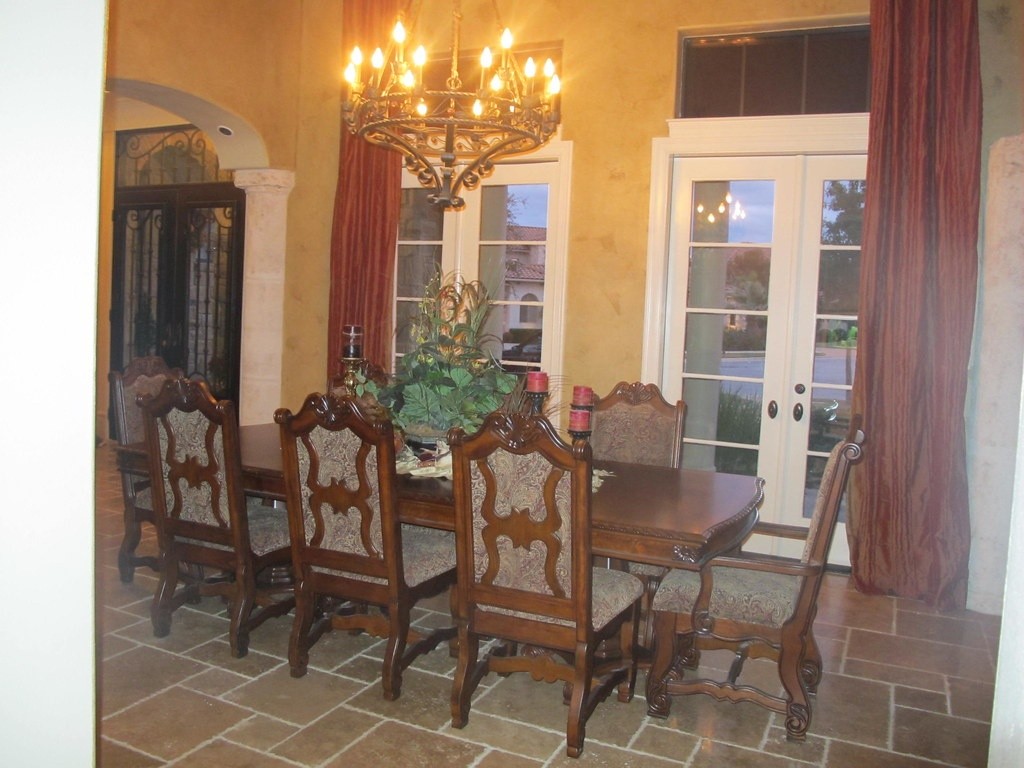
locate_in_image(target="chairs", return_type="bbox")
[642,408,865,742]
[271,392,459,703]
[590,380,687,589]
[107,357,192,581]
[445,406,645,759]
[133,374,322,658]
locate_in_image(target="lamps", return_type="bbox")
[342,1,565,213]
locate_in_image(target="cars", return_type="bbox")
[502,321,541,374]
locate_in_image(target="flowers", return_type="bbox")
[354,263,520,429]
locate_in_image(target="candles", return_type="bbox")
[527,371,593,434]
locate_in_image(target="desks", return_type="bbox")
[229,422,767,703]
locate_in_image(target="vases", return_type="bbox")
[404,435,451,468]
